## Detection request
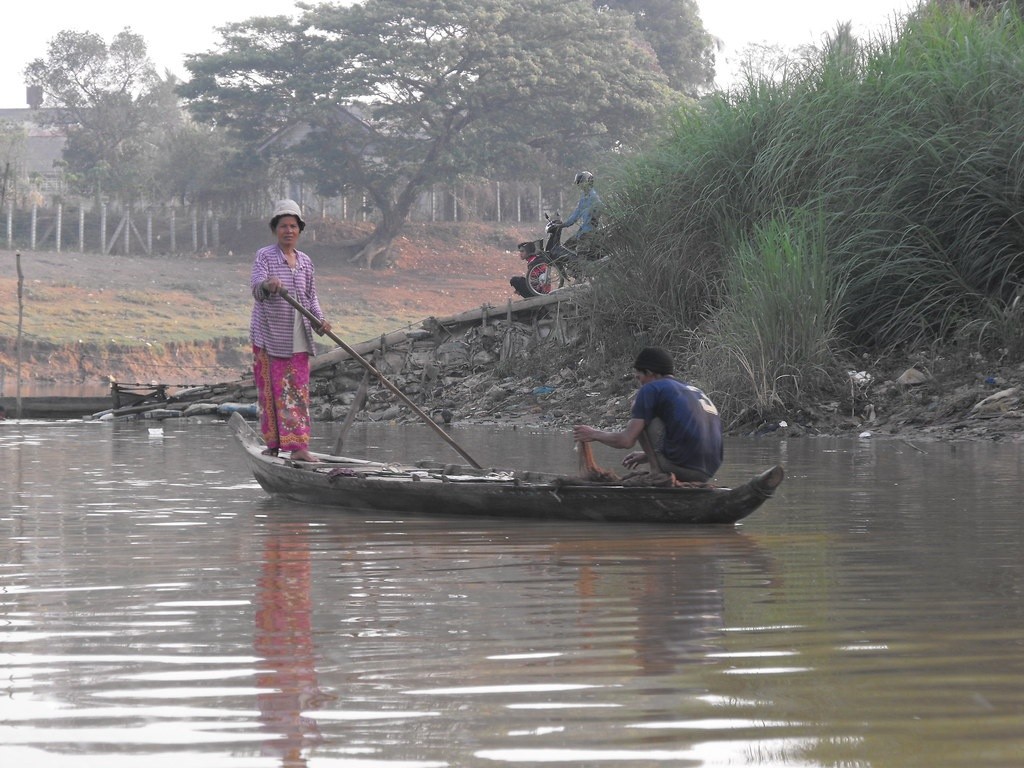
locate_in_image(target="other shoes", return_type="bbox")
[623,473,672,488]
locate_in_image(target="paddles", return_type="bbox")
[276,283,485,472]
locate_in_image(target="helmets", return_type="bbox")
[573,171,594,186]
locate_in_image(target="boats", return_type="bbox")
[228,411,784,523]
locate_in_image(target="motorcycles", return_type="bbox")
[527,207,625,296]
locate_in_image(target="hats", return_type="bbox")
[270,199,306,228]
[636,347,674,373]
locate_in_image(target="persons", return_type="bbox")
[574,347,723,482]
[559,171,604,259]
[510,242,551,298]
[250,199,332,462]
